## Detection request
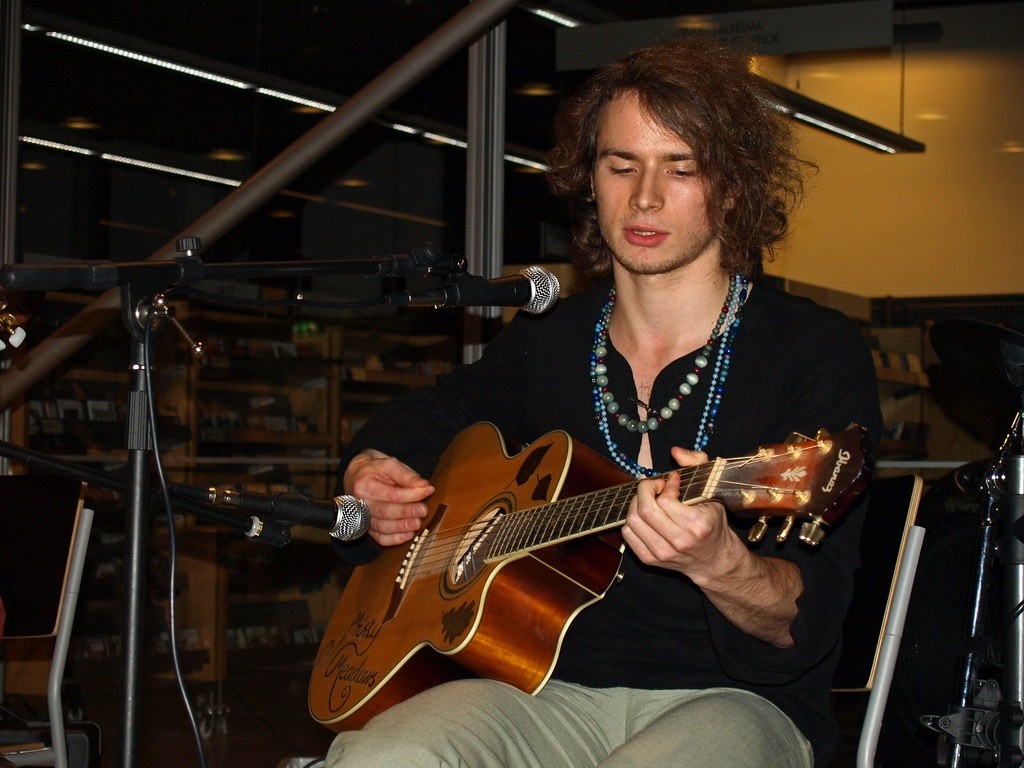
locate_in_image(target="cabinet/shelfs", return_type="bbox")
[0,262,581,768]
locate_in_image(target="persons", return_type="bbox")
[314,33,882,768]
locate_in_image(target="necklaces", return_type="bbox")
[588,272,749,485]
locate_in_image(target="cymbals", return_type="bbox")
[929,315,1024,409]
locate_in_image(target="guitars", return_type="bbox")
[306,419,881,729]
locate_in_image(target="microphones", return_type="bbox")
[206,487,371,541]
[388,265,561,313]
